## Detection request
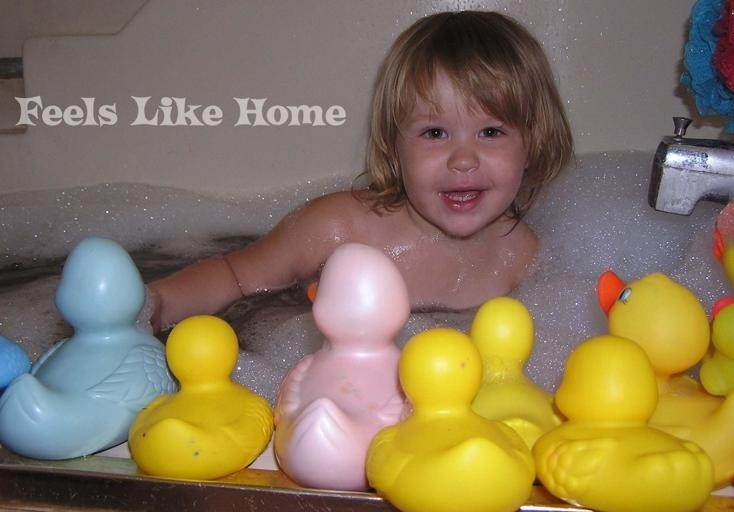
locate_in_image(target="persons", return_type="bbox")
[133,10,575,337]
[594,204,734,490]
